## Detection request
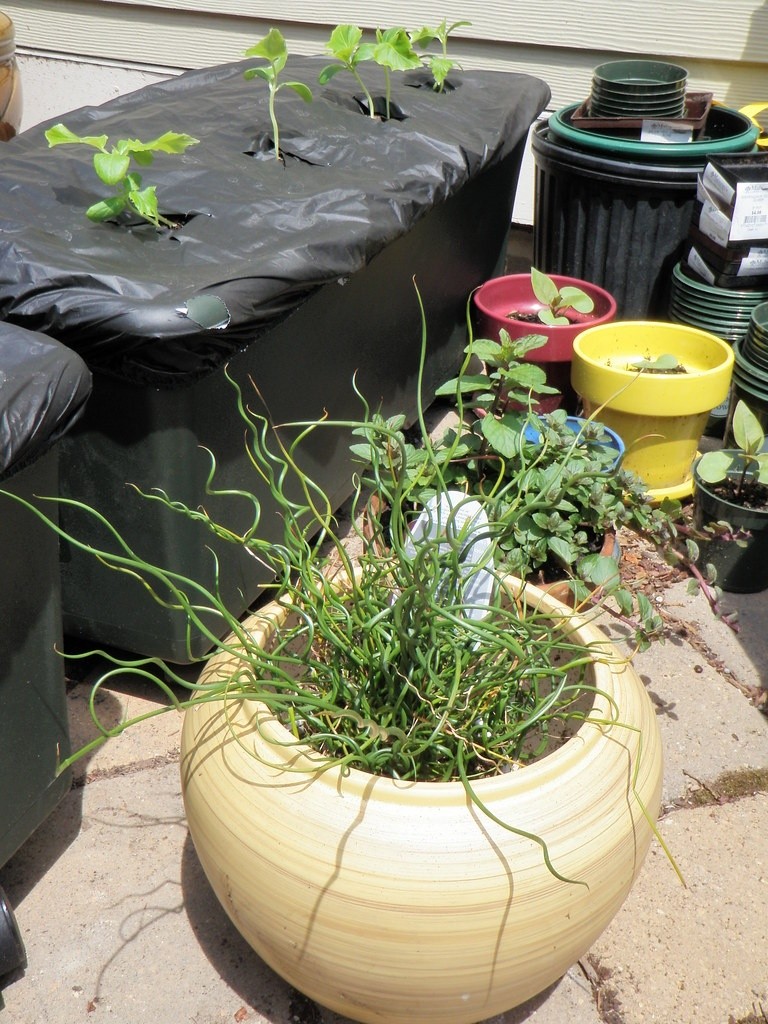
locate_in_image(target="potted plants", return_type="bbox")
[0,53,550,667]
[1,263,766,1024]
